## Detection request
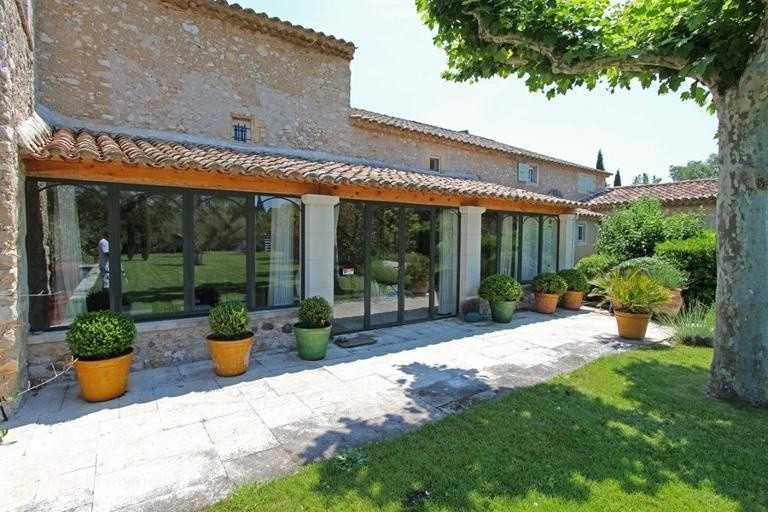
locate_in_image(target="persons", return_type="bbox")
[97,229,111,308]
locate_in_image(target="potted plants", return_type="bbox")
[294,293,331,362]
[204,299,259,378]
[588,266,688,342]
[480,270,522,323]
[405,251,430,298]
[65,312,140,403]
[531,268,587,314]
